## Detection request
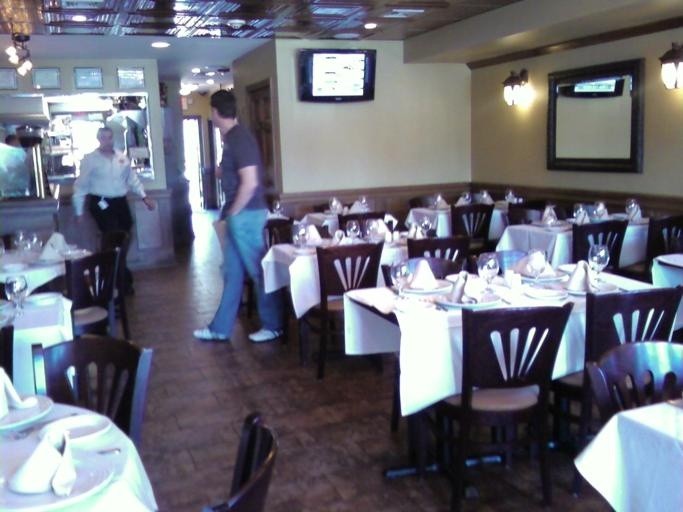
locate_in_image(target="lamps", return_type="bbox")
[659,42,683,90]
[5,21,33,77]
[502,68,528,106]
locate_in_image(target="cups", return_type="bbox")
[588,245,609,292]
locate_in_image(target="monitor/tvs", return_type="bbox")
[558,76,625,98]
[297,49,376,103]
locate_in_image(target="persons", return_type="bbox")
[192,89,285,342]
[4,126,26,146]
[71,127,156,295]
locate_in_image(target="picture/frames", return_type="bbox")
[32,66,60,90]
[117,66,145,89]
[0,66,17,90]
[73,67,103,89]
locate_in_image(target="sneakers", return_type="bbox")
[248,328,284,342]
[193,328,227,340]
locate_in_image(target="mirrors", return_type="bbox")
[547,55,645,173]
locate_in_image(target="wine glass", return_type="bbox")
[391,264,414,315]
[272,201,282,218]
[328,196,338,216]
[572,203,586,225]
[479,190,488,203]
[292,247,318,255]
[366,219,379,244]
[596,203,607,222]
[506,190,514,203]
[28,232,42,265]
[14,231,25,262]
[5,275,27,323]
[19,240,32,269]
[543,203,557,232]
[478,253,500,297]
[421,216,431,237]
[463,193,472,205]
[293,223,310,253]
[359,196,366,214]
[626,200,637,225]
[434,193,442,209]
[347,220,361,244]
[0,240,5,273]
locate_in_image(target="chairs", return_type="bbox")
[407,235,465,268]
[381,257,460,286]
[32,336,153,452]
[409,195,432,208]
[0,325,14,385]
[338,210,386,238]
[246,216,294,319]
[203,411,280,512]
[585,341,683,417]
[508,200,544,225]
[624,215,683,283]
[451,204,489,273]
[415,303,574,512]
[549,285,683,496]
[477,239,502,275]
[573,220,628,275]
[299,240,384,379]
[100,231,132,340]
[65,247,121,338]
[313,202,352,212]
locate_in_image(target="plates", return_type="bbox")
[24,292,61,305]
[3,263,24,273]
[528,288,569,303]
[38,414,112,443]
[435,291,486,308]
[512,269,568,285]
[568,218,587,224]
[0,450,115,510]
[564,282,619,297]
[0,393,54,430]
[400,278,453,293]
[63,249,84,257]
[532,218,566,227]
